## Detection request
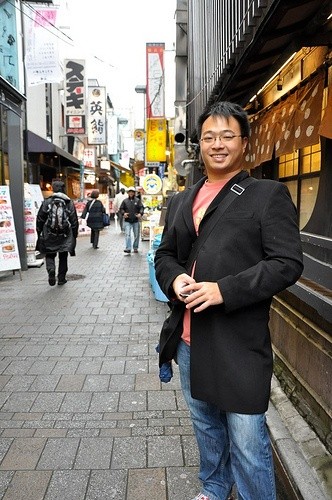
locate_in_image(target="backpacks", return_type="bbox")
[41,196,71,243]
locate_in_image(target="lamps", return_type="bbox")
[135,84,146,93]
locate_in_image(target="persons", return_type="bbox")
[154,102,305,499]
[119,187,145,254]
[35,179,79,286]
[113,187,129,233]
[80,191,108,249]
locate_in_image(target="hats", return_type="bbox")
[129,186,136,191]
[53,181,65,187]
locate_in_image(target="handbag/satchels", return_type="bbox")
[102,213,111,226]
[83,199,95,226]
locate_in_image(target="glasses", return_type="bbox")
[200,133,241,144]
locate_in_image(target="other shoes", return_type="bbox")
[49,269,55,286]
[134,249,138,253]
[190,492,210,500]
[124,249,131,253]
[93,244,99,249]
[58,279,67,285]
